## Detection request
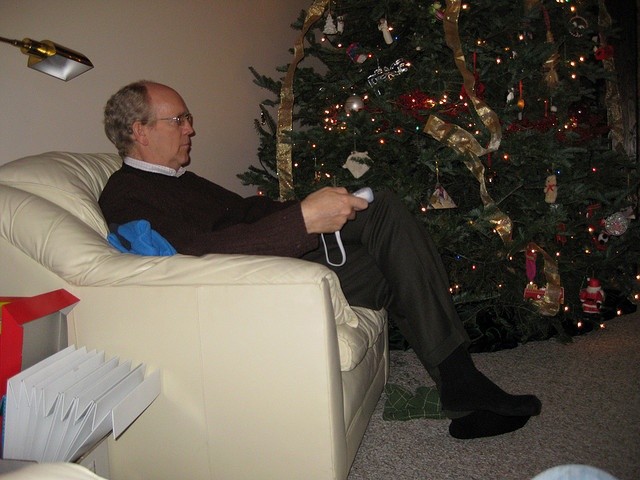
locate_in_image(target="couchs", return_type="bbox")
[0,152,390,480]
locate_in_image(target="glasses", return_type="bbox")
[137,114,194,126]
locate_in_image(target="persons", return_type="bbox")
[98,79,543,440]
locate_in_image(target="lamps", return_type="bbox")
[0,36,94,82]
